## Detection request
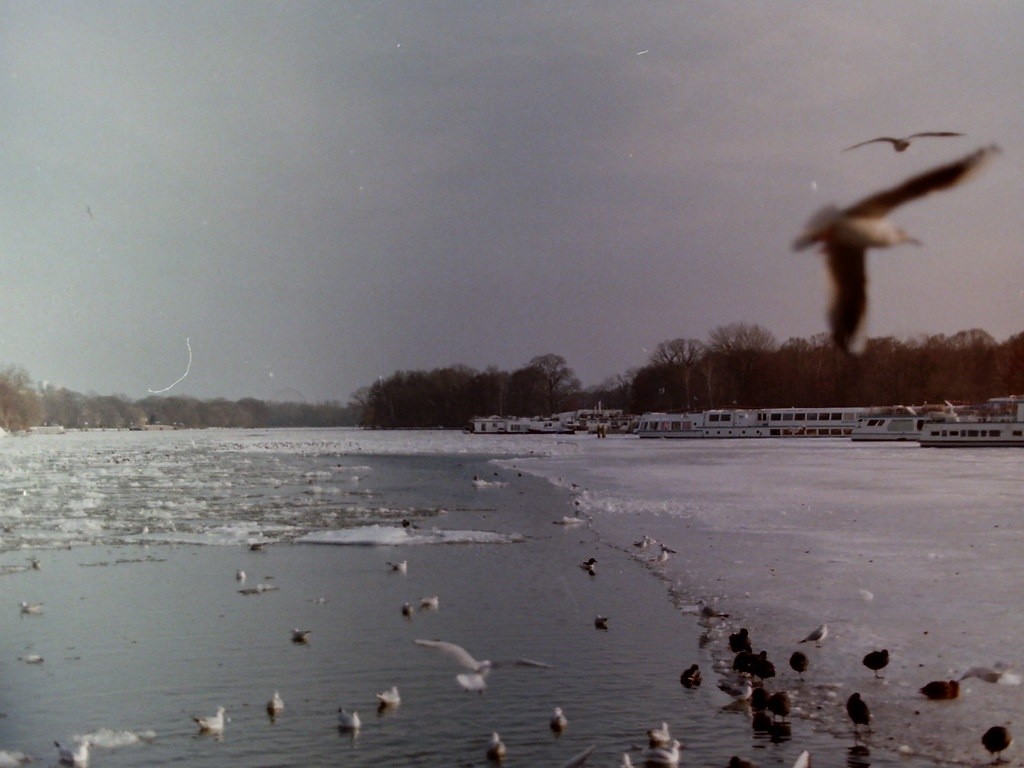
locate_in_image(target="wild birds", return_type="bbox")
[861,649,890,679]
[798,623,827,648]
[981,726,1012,760]
[385,560,438,617]
[248,542,266,551]
[412,639,555,694]
[847,693,872,732]
[633,535,677,562]
[581,558,598,576]
[680,663,702,690]
[487,708,810,768]
[795,144,986,355]
[54,738,95,763]
[846,131,964,151]
[696,600,729,619]
[193,685,400,730]
[594,615,610,629]
[917,680,959,699]
[290,628,311,645]
[717,628,810,722]
